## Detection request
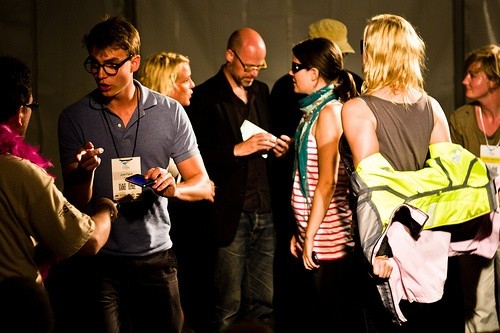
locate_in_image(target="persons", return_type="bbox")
[271,20,374,333]
[138,51,196,110]
[448,44,500,333]
[184,29,294,333]
[289,38,361,333]
[59,14,211,333]
[0,57,121,333]
[340,13,496,333]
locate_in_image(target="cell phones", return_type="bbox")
[126,174,157,188]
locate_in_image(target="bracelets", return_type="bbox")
[96,197,119,223]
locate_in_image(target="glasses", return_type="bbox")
[291,61,306,73]
[83,54,133,76]
[25,101,40,112]
[230,48,268,71]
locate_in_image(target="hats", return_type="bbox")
[306,17,356,53]
